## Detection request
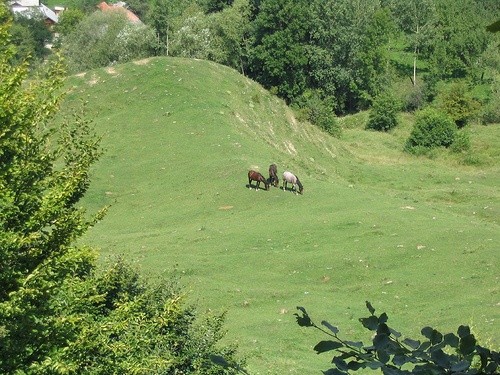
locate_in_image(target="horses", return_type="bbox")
[248,169,270,190]
[269,162,279,186]
[282,170,305,195]
